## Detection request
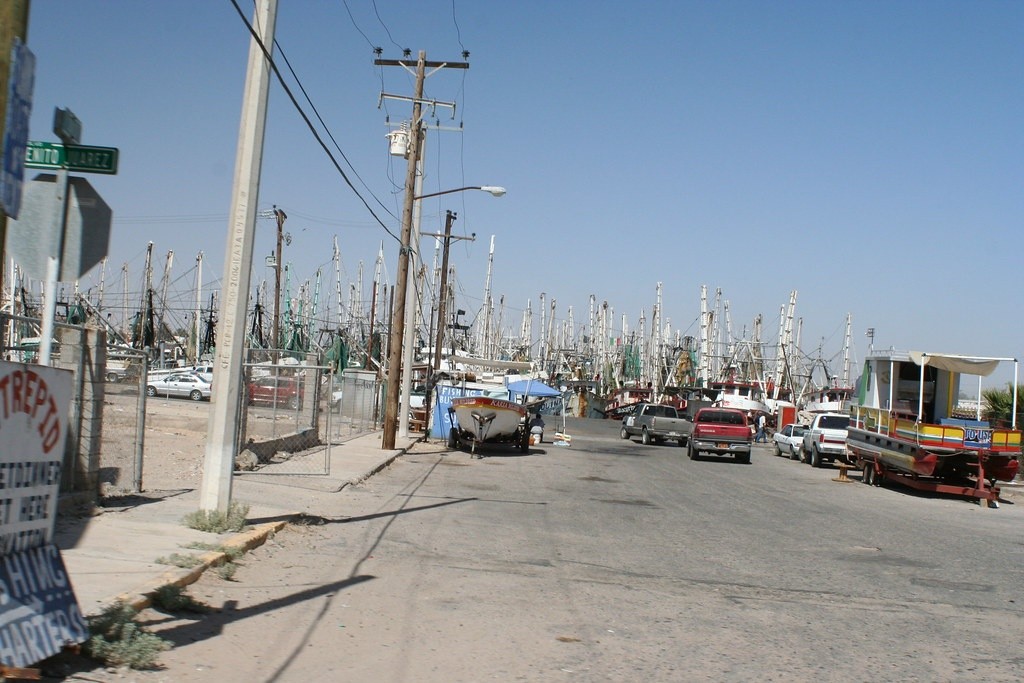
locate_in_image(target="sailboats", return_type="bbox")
[4,230,500,392]
[508,282,855,420]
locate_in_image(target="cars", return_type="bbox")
[772,411,851,467]
[145,364,214,402]
[246,357,426,428]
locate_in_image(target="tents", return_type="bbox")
[508,379,566,434]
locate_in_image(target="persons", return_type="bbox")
[754,412,768,443]
[530,414,545,442]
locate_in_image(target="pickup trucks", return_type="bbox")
[105,367,127,382]
[686,408,754,462]
[619,404,691,445]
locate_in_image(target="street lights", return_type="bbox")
[380,188,508,450]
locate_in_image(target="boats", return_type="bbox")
[450,396,525,441]
